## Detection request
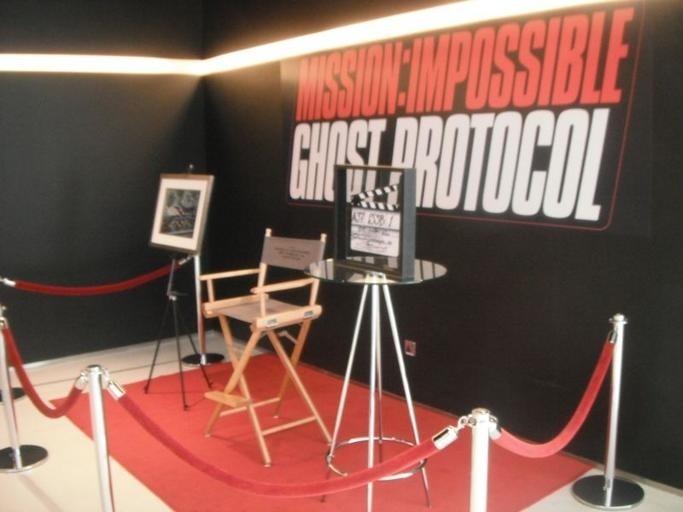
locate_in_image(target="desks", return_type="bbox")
[304,256,447,512]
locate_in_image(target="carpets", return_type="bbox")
[49,352,599,512]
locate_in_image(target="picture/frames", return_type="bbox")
[147,172,214,254]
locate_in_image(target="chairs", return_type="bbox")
[198,227,333,468]
